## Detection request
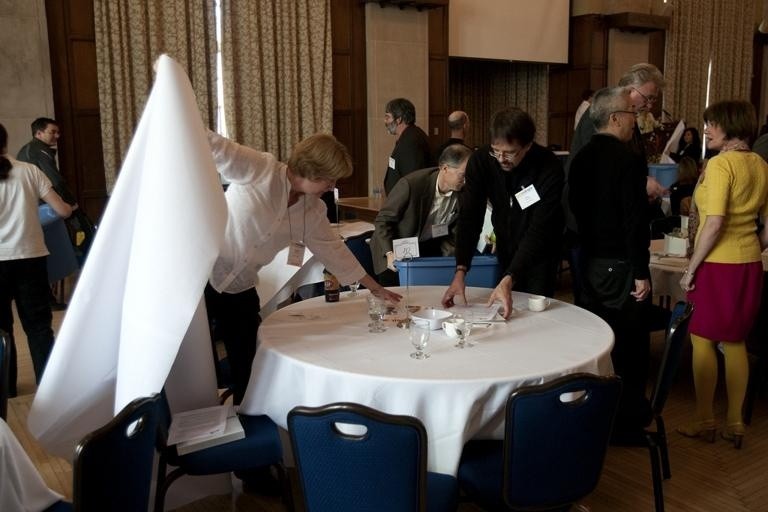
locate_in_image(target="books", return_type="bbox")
[177,404,246,456]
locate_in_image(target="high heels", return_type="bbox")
[721,422,746,450]
[675,415,717,443]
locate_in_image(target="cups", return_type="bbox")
[453,311,473,349]
[408,320,431,360]
[441,319,465,338]
[527,296,550,312]
[367,294,386,333]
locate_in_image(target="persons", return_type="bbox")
[0,124,73,399]
[574,90,597,131]
[434,110,475,166]
[569,63,669,428]
[371,145,497,287]
[674,99,768,449]
[384,98,434,197]
[443,107,566,321]
[15,117,68,310]
[665,127,701,228]
[567,86,662,447]
[151,53,404,406]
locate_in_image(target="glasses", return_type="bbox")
[612,110,638,115]
[633,87,657,103]
[488,150,521,159]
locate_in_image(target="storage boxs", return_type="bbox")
[647,161,679,197]
[31,203,79,282]
[393,256,501,288]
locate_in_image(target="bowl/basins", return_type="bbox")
[411,309,453,331]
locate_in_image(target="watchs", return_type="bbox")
[684,269,694,275]
[454,269,467,274]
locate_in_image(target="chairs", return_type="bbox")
[280,403,454,512]
[157,385,281,512]
[43,393,164,512]
[612,299,692,512]
[462,372,615,512]
